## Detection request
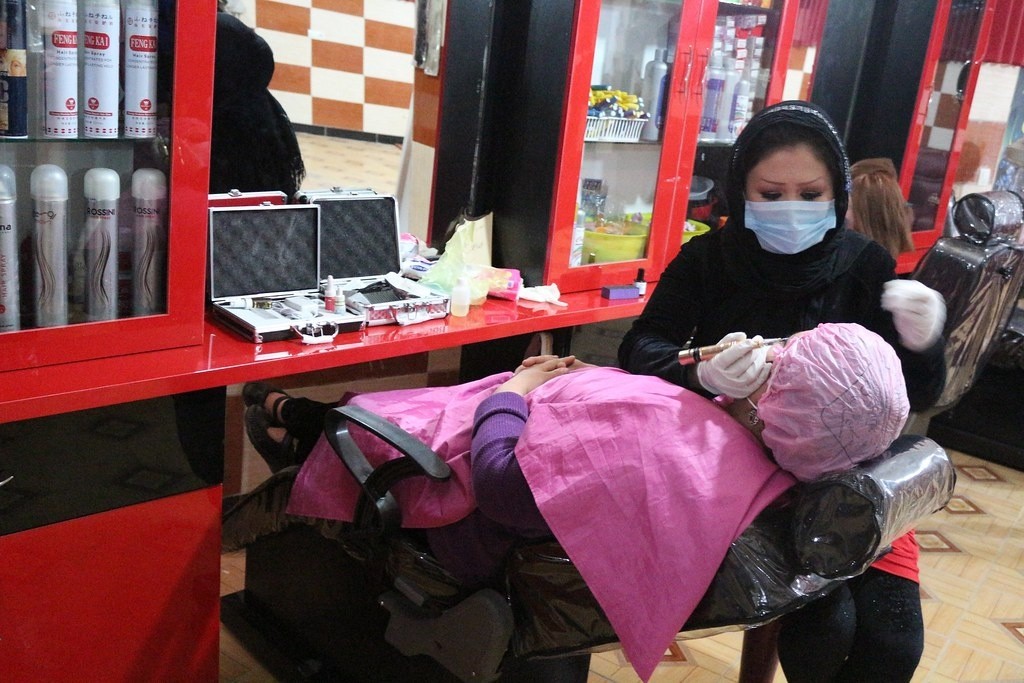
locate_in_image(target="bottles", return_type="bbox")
[30,164,68,326]
[230,297,272,310]
[0,0,28,138]
[324,275,336,310]
[640,48,749,142]
[124,0,159,138]
[632,268,647,296]
[334,287,346,314]
[45,0,79,139]
[83,167,120,320]
[568,202,585,267]
[0,164,20,331]
[451,277,471,317]
[130,168,167,317]
[84,0,119,139]
[588,253,596,263]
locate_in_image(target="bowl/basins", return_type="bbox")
[684,175,714,221]
[581,212,711,267]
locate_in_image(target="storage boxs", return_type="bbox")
[312,195,450,325]
[210,204,366,345]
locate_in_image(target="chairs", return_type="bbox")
[221,405,953,681]
[900,191,1024,438]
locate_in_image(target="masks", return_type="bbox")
[743,187,837,254]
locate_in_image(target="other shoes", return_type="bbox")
[241,381,292,426]
[244,404,295,471]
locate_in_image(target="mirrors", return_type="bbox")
[898,0,988,254]
[209,0,454,270]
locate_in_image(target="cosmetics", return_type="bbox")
[0,1,157,138]
[83,168,121,322]
[324,275,346,316]
[643,14,768,142]
[131,168,167,315]
[30,164,69,327]
[568,211,586,268]
[0,163,20,331]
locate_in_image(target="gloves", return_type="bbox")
[881,280,947,350]
[696,331,772,399]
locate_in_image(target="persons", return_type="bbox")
[242,321,911,577]
[844,158,916,259]
[208,0,306,198]
[617,100,948,683]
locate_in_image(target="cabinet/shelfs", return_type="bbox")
[470,0,801,296]
[0,0,214,375]
[1,383,222,682]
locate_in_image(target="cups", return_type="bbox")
[750,68,770,119]
